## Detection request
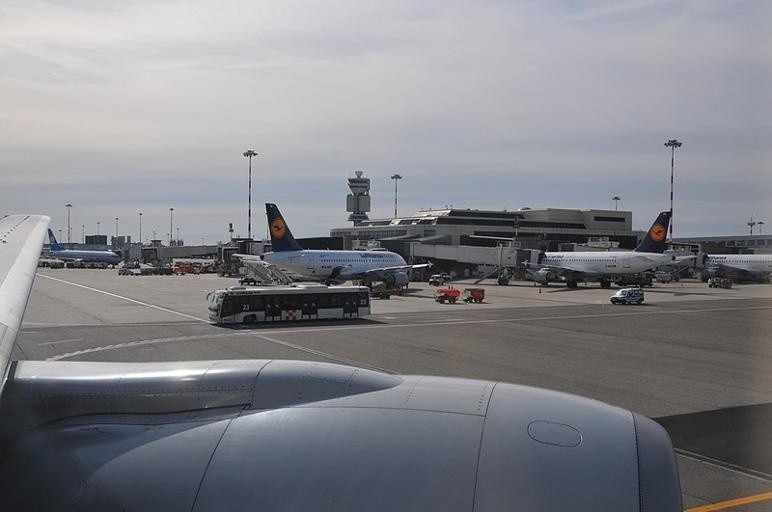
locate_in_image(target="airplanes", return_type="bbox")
[47,227,121,267]
[698,250,771,282]
[0,211,682,510]
[233,201,431,289]
[525,211,695,288]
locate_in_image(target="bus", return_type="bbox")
[204,281,373,326]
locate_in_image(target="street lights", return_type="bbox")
[61,199,181,244]
[661,138,681,239]
[748,217,756,235]
[758,221,764,235]
[390,171,404,220]
[612,195,620,210]
[241,149,258,238]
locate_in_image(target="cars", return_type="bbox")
[429,274,444,286]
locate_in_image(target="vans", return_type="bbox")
[610,288,645,304]
